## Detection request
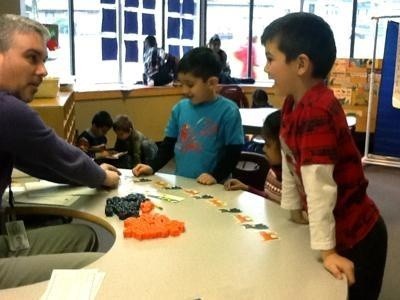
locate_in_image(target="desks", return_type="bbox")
[0,163,348,299]
[231,106,358,133]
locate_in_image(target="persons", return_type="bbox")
[220,109,282,207]
[260,10,389,299]
[134,35,167,86]
[206,35,231,82]
[231,33,260,78]
[74,111,131,170]
[131,46,247,186]
[250,88,273,108]
[112,116,159,168]
[0,12,123,289]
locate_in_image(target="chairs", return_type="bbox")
[250,86,274,109]
[218,85,267,155]
[228,149,273,194]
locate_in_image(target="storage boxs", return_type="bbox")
[32,76,62,99]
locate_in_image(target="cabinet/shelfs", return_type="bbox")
[22,90,79,149]
